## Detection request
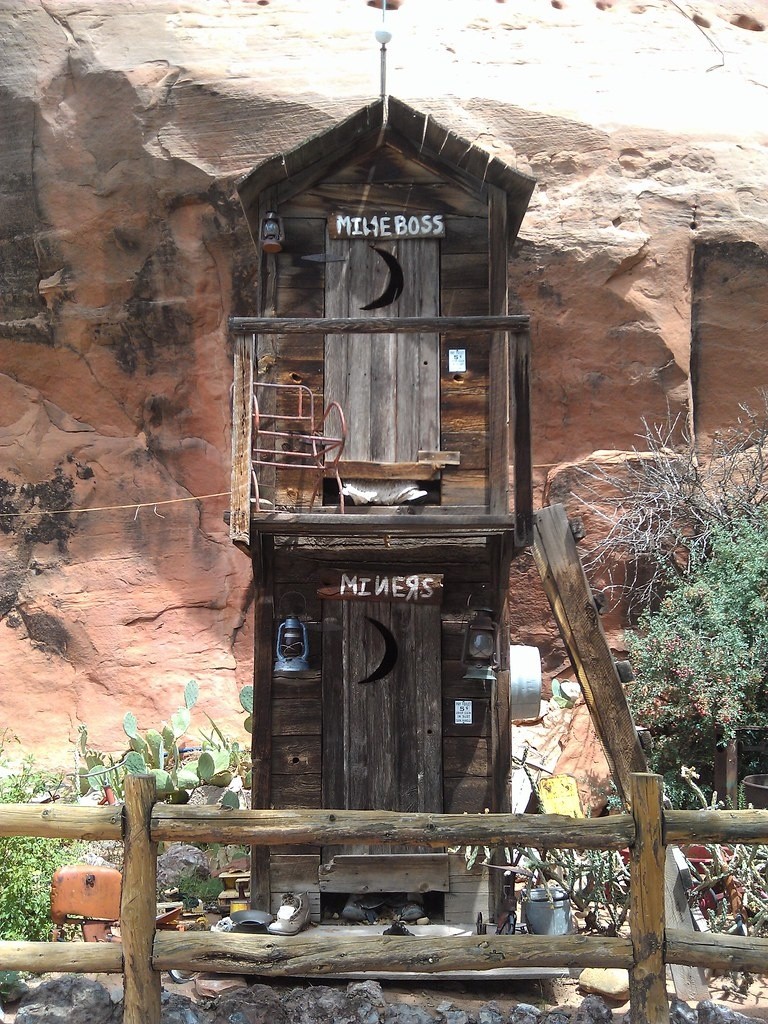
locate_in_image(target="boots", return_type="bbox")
[267,892,310,935]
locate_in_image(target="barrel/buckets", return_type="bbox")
[742,774,768,809]
[522,888,573,935]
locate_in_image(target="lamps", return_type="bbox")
[273,590,310,672]
[259,210,285,254]
[459,585,500,680]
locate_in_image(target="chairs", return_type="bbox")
[229,381,348,514]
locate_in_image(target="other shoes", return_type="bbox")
[402,904,424,921]
[344,905,373,921]
